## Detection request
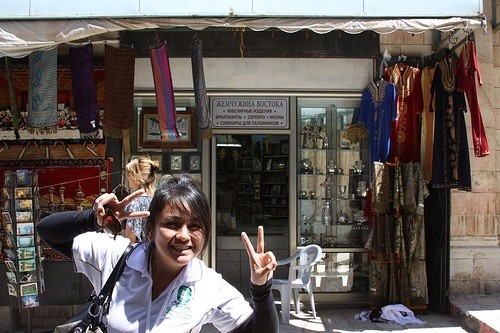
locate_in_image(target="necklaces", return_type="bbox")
[146,249,180,333]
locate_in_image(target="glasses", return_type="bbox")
[93,195,123,240]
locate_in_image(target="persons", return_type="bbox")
[125,156,159,243]
[36,174,280,333]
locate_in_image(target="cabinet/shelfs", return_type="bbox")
[297,147,368,248]
[233,156,289,221]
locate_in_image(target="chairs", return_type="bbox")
[271,244,323,325]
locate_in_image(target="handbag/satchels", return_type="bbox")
[53,297,106,333]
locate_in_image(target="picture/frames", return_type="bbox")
[136,106,198,149]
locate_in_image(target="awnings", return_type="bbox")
[0,13,488,60]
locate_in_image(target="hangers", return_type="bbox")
[374,74,381,83]
[446,52,453,59]
[398,57,407,72]
